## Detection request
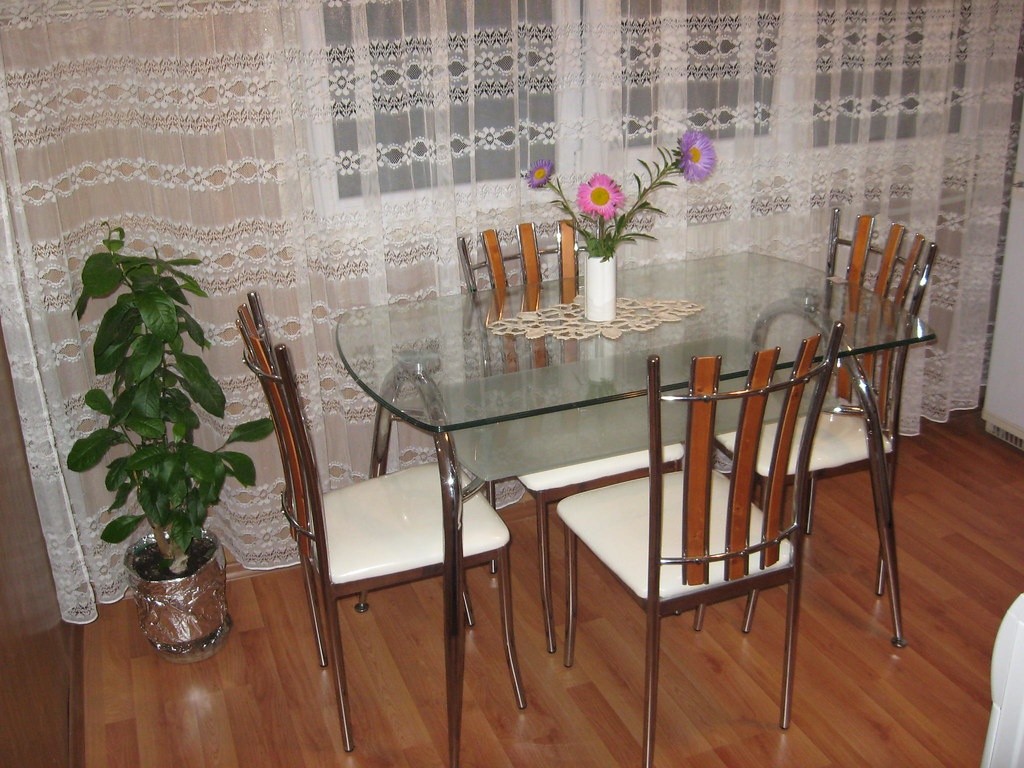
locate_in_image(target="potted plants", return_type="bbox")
[62,219,274,662]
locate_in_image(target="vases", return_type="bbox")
[583,256,617,322]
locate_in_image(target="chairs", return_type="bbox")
[236,291,525,754]
[547,320,842,768]
[712,208,938,635]
[457,217,687,653]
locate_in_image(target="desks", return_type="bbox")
[335,251,937,768]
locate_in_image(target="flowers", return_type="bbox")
[526,131,717,262]
